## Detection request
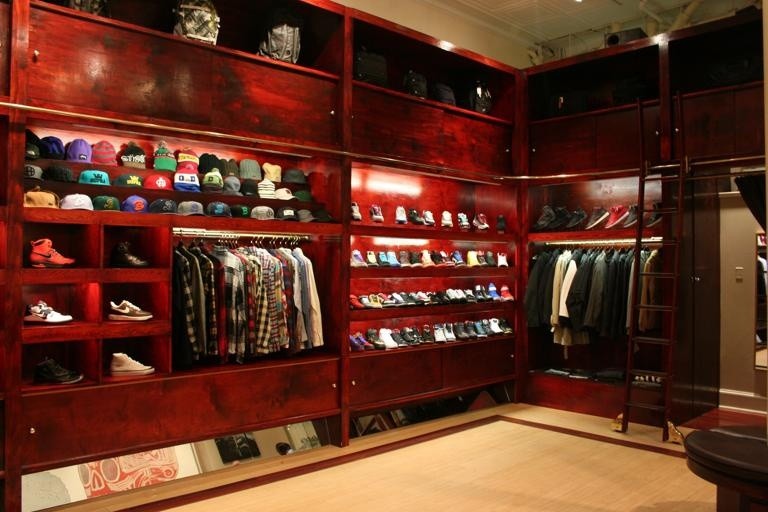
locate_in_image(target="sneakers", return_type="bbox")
[489,317,504,335]
[477,252,488,266]
[443,323,456,341]
[487,283,501,301]
[454,289,467,304]
[390,328,409,347]
[604,205,631,229]
[395,206,408,224]
[409,251,423,268]
[565,208,587,229]
[359,295,374,309]
[349,295,366,310]
[106,299,154,321]
[365,251,380,266]
[408,292,424,305]
[472,213,490,230]
[623,204,648,228]
[408,208,425,225]
[370,205,385,222]
[33,359,84,384]
[350,250,368,267]
[422,324,434,344]
[499,319,513,334]
[457,213,470,228]
[419,250,436,268]
[410,326,425,344]
[431,250,448,266]
[464,320,477,338]
[473,321,488,338]
[386,251,400,267]
[451,251,465,265]
[464,289,478,304]
[24,302,73,326]
[533,205,555,231]
[378,252,390,266]
[466,250,481,266]
[496,252,509,268]
[486,251,497,266]
[432,324,447,342]
[547,207,570,230]
[495,215,506,231]
[426,292,444,306]
[377,293,401,308]
[107,241,148,268]
[481,285,494,301]
[441,211,454,228]
[368,293,382,309]
[378,328,399,349]
[399,251,412,268]
[482,319,494,336]
[365,329,386,350]
[434,291,451,305]
[27,238,77,269]
[445,288,460,305]
[349,334,365,352]
[454,322,470,339]
[400,327,421,346]
[500,285,515,302]
[391,293,407,308]
[353,332,375,351]
[422,210,436,225]
[416,291,432,306]
[439,250,455,266]
[584,207,610,230]
[474,284,486,302]
[645,201,663,228]
[109,352,155,377]
[351,202,363,221]
[398,292,416,306]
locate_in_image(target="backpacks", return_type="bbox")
[168,0,221,46]
[404,69,428,98]
[354,42,388,87]
[69,0,112,18]
[256,9,302,64]
[467,78,497,114]
[433,79,456,107]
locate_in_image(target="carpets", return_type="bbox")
[679,406,767,430]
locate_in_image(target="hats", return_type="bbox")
[239,159,262,178]
[293,190,314,203]
[298,209,320,224]
[198,153,225,176]
[66,138,92,163]
[176,201,206,216]
[143,174,174,191]
[24,164,46,182]
[41,165,76,184]
[262,162,282,182]
[224,176,244,196]
[153,140,178,173]
[176,146,200,174]
[23,186,59,208]
[59,193,94,210]
[26,128,40,159]
[120,194,151,213]
[231,204,251,218]
[149,198,178,216]
[277,206,300,221]
[312,209,338,223]
[240,181,258,196]
[201,167,224,193]
[257,179,278,199]
[112,173,143,188]
[76,169,111,187]
[173,168,201,193]
[205,202,233,218]
[219,159,239,177]
[283,169,307,184]
[276,187,298,201]
[39,136,65,160]
[121,141,146,170]
[91,140,117,166]
[250,206,278,221]
[91,195,121,211]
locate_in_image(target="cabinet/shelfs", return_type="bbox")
[2,0,350,157]
[348,154,526,441]
[1,105,347,511]
[343,2,521,185]
[523,177,662,428]
[700,192,768,416]
[520,35,666,174]
[668,10,767,163]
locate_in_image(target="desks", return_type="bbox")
[684,424,767,511]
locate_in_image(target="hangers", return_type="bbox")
[532,242,661,267]
[174,231,303,255]
